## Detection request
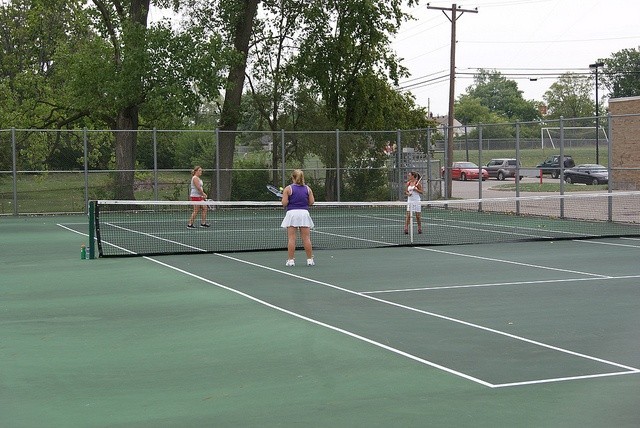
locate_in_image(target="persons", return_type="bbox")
[187,166,210,229]
[282,169,316,267]
[404,171,422,234]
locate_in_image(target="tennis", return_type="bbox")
[279,187,283,192]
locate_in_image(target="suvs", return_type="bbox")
[481,158,523,181]
[536,154,575,179]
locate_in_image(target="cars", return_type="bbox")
[563,164,609,185]
[441,162,489,181]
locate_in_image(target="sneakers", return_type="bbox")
[418,229,422,234]
[200,223,210,228]
[306,255,315,266]
[405,229,408,234]
[187,223,198,230]
[285,258,295,266]
